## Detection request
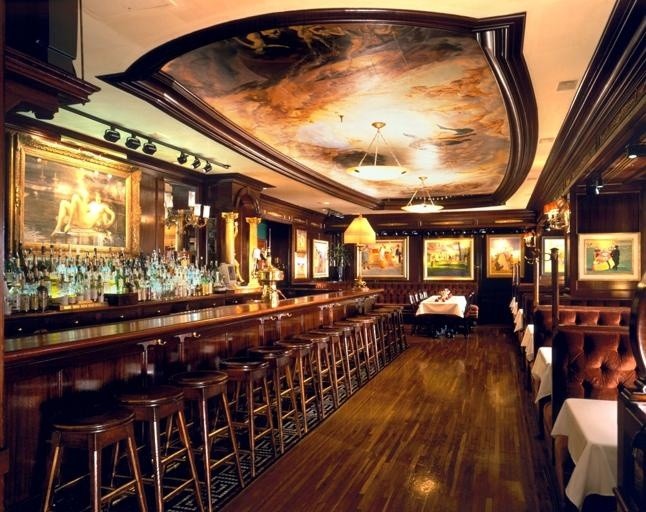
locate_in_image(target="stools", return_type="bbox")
[246,345,303,440]
[272,338,320,422]
[208,356,278,478]
[324,305,409,397]
[107,382,206,512]
[294,333,338,409]
[39,402,149,512]
[161,370,245,512]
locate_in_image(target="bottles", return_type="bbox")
[16,272,24,289]
[29,286,37,310]
[101,245,220,301]
[67,275,75,304]
[19,287,29,311]
[5,249,14,269]
[95,257,103,273]
[90,274,96,303]
[12,267,18,282]
[32,253,38,279]
[47,255,55,273]
[75,274,83,304]
[47,244,56,264]
[86,261,94,273]
[73,246,81,261]
[83,274,90,303]
[23,248,26,261]
[65,244,71,257]
[13,255,24,285]
[14,249,20,268]
[26,248,34,260]
[49,266,59,296]
[41,266,50,299]
[54,255,64,273]
[26,260,33,283]
[70,257,76,274]
[91,248,99,261]
[5,269,12,282]
[37,276,48,311]
[63,256,69,282]
[79,259,86,275]
[38,246,47,270]
[83,250,90,266]
[7,286,19,310]
[96,274,103,303]
[92,256,97,269]
[54,248,63,272]
[74,254,79,265]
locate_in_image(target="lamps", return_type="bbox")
[142,138,157,156]
[347,121,410,181]
[544,201,564,230]
[191,158,201,170]
[626,138,640,160]
[201,161,212,174]
[176,152,190,165]
[124,132,141,150]
[102,126,121,143]
[584,175,606,197]
[343,212,376,292]
[400,175,444,213]
[522,232,537,248]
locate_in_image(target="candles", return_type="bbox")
[188,191,195,207]
[193,204,200,216]
[203,205,210,218]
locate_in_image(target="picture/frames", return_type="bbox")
[295,229,307,253]
[487,235,526,278]
[577,232,642,280]
[354,236,410,281]
[421,236,478,282]
[541,235,565,278]
[312,239,330,278]
[293,252,309,280]
[7,129,142,261]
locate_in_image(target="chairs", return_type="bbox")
[408,288,428,338]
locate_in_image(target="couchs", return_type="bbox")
[555,323,638,512]
[534,305,632,456]
